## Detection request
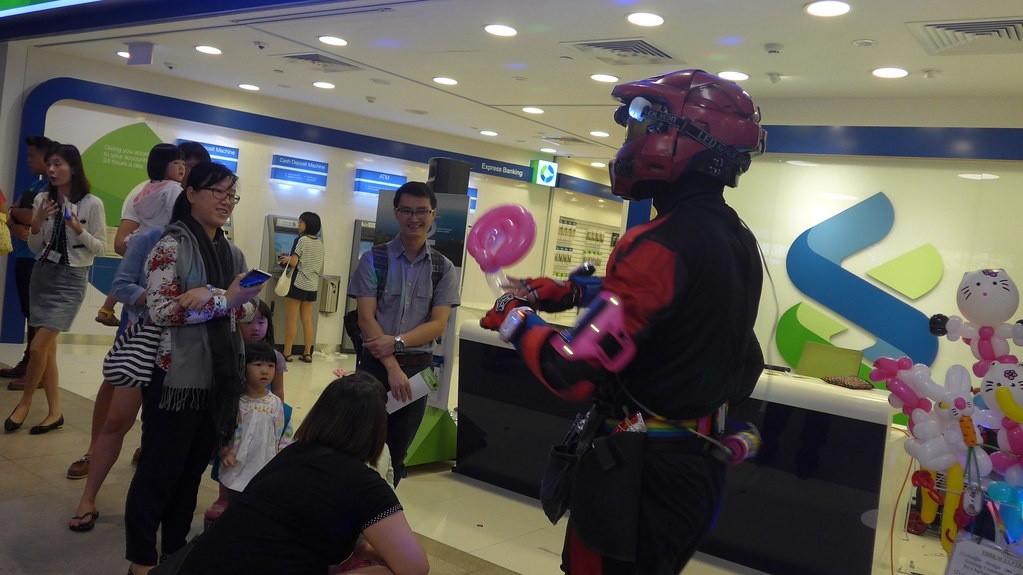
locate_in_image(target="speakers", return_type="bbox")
[427,157,470,195]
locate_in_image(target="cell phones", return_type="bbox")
[240,268,272,287]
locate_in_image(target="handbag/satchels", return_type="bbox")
[274,256,295,298]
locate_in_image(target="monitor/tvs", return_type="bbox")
[275,232,298,261]
[358,241,373,260]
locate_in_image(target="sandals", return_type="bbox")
[95,308,120,326]
[298,353,312,363]
[282,353,294,362]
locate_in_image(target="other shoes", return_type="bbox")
[67,454,93,479]
[127,564,157,575]
[204,498,228,521]
[30,413,64,434]
[131,448,142,465]
[4,404,29,432]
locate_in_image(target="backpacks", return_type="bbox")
[344,243,445,367]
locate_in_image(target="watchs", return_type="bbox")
[394,335,405,353]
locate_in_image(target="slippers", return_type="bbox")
[69,511,99,532]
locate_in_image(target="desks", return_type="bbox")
[452,324,915,575]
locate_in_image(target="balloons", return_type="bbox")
[869,267,1023,557]
[466,206,536,299]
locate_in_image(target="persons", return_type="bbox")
[479,70,765,575]
[0,134,461,575]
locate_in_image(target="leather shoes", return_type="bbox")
[8,380,45,391]
[0,361,27,378]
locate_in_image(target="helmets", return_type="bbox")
[608,68,767,202]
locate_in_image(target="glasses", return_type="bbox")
[198,187,240,204]
[396,208,434,217]
